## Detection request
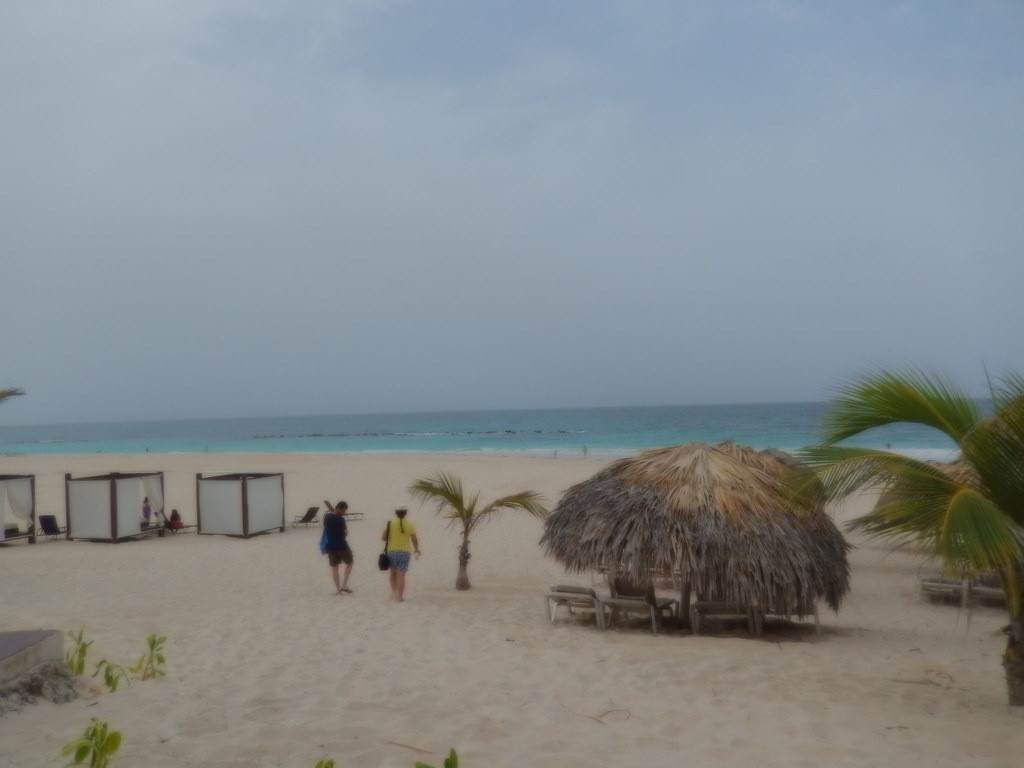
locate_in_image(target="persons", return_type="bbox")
[167,509,183,535]
[142,496,151,527]
[382,502,420,602]
[322,500,354,596]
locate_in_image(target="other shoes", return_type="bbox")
[336,591,342,596]
[341,586,352,593]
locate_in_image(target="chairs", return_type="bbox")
[36,515,67,541]
[160,509,198,535]
[543,584,600,629]
[691,580,822,638]
[594,563,682,635]
[916,571,1008,605]
[323,501,364,520]
[286,506,321,529]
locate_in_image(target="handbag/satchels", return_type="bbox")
[319,529,328,554]
[378,553,390,570]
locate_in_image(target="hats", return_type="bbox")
[392,503,409,510]
